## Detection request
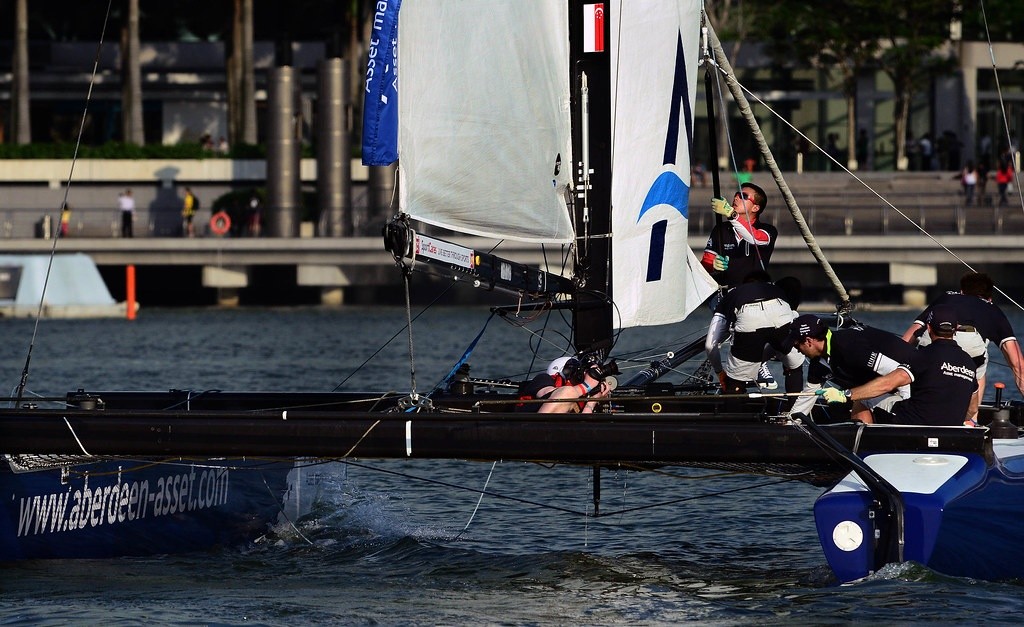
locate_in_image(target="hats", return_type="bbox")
[791,313,828,343]
[961,272,995,300]
[927,303,960,332]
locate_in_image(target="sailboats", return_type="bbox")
[0,0,1024,585]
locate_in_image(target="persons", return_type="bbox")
[61,202,70,237]
[961,129,1019,207]
[120,188,136,238]
[816,305,979,426]
[785,314,917,426]
[181,187,195,236]
[514,357,609,413]
[705,283,806,401]
[907,130,961,171]
[199,133,228,154]
[700,183,778,389]
[901,272,1024,426]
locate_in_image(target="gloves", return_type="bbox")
[821,386,848,403]
[710,195,734,218]
[712,253,731,271]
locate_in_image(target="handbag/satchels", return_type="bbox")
[1007,182,1013,194]
[708,291,728,316]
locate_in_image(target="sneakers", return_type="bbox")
[752,361,778,390]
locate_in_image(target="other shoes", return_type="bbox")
[717,370,731,393]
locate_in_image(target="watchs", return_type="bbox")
[727,211,737,220]
[844,388,852,402]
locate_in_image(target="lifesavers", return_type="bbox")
[210,212,231,234]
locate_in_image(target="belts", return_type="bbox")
[749,298,767,302]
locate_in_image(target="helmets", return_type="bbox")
[547,355,582,383]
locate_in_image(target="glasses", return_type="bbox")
[733,191,754,207]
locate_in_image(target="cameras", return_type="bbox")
[598,358,623,383]
